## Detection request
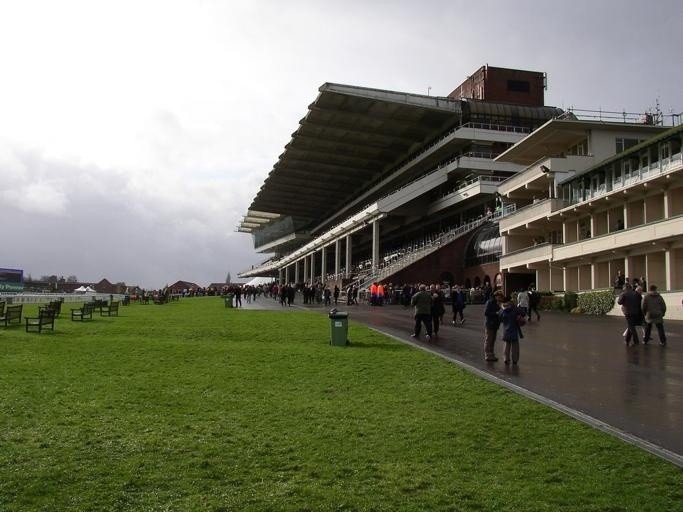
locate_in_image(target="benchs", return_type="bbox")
[37,300,120,322]
[0,300,4,314]
[0,305,23,327]
[25,310,54,334]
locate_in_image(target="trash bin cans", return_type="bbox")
[328,311,350,346]
[224,295,233,308]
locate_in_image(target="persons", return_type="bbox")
[409,283,445,342]
[622,286,653,343]
[302,279,331,306]
[511,284,542,323]
[618,281,653,347]
[346,285,354,305]
[367,280,493,306]
[485,206,491,214]
[106,293,114,306]
[616,219,623,230]
[642,284,667,347]
[449,284,466,326]
[351,284,359,305]
[123,283,295,309]
[333,285,340,305]
[532,195,539,204]
[531,237,540,246]
[482,289,503,362]
[498,296,525,365]
[614,268,647,293]
